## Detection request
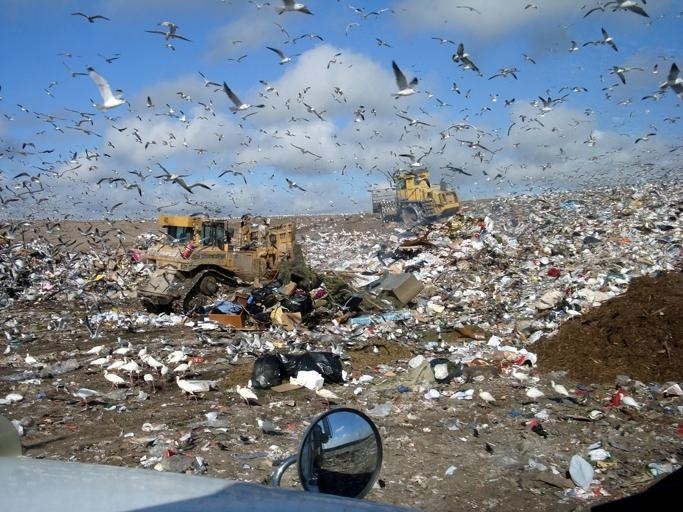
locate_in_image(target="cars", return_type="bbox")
[0,402,447,512]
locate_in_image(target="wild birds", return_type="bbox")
[0,0,683,414]
[255,416,283,435]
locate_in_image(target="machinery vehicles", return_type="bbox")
[133,210,313,316]
[377,162,463,230]
